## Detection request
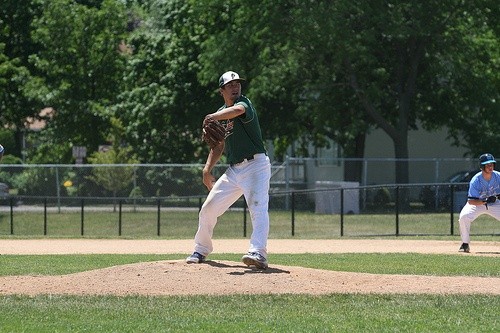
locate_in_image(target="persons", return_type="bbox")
[458,154,500,251]
[186,70,271,270]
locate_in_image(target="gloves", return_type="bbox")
[482,194,500,210]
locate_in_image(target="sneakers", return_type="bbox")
[186,251,205,263]
[458,242,470,253]
[241,251,268,270]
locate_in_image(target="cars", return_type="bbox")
[420,169,481,209]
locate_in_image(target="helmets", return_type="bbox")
[479,153,496,164]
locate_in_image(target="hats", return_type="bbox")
[218,71,245,87]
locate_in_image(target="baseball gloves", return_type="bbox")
[201,119,227,147]
[466,196,490,206]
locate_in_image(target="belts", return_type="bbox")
[237,155,254,163]
